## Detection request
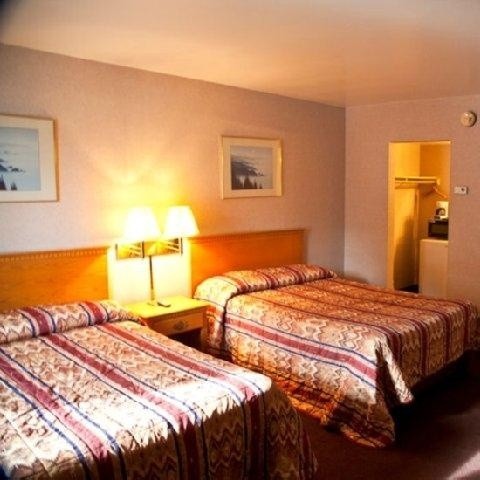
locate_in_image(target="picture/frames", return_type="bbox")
[219,134,284,200]
[0,113,61,204]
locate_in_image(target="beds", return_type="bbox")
[0,247,326,480]
[188,227,480,449]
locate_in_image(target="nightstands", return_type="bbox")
[125,295,210,355]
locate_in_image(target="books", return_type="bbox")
[434,200,448,221]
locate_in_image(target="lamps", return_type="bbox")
[120,207,160,242]
[162,207,199,240]
